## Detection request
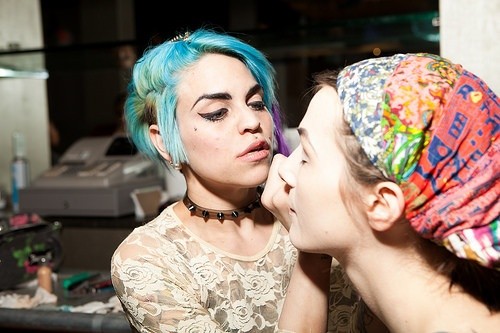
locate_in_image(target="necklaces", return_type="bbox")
[183,185,265,220]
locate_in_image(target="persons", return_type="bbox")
[275,52,500,333]
[110,27,392,333]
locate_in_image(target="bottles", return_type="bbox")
[37,257,54,295]
[11,134,30,213]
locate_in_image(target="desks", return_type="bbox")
[0,268,134,333]
